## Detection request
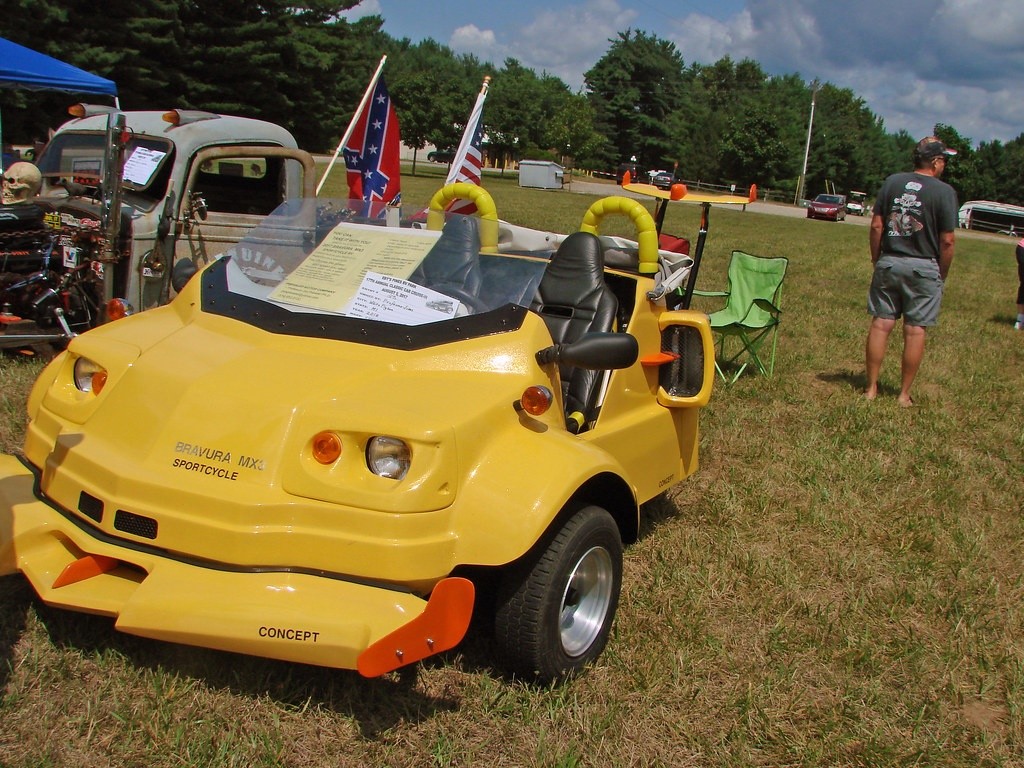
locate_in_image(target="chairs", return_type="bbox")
[510,231,619,433]
[682,251,790,388]
[407,216,484,315]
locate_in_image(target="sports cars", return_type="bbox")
[0,183,717,691]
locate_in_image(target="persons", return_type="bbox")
[865,135,959,405]
[1015,236,1023,331]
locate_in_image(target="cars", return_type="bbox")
[847,198,866,216]
[27,102,317,320]
[427,146,458,164]
[654,172,681,190]
[807,194,847,221]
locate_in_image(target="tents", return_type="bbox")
[0,38,121,109]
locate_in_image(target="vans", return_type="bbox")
[617,163,650,185]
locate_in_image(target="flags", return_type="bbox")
[409,88,488,222]
[343,72,402,226]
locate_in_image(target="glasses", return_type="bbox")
[930,156,948,163]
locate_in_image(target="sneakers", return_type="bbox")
[1014,321,1024,329]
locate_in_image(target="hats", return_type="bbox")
[914,138,957,155]
